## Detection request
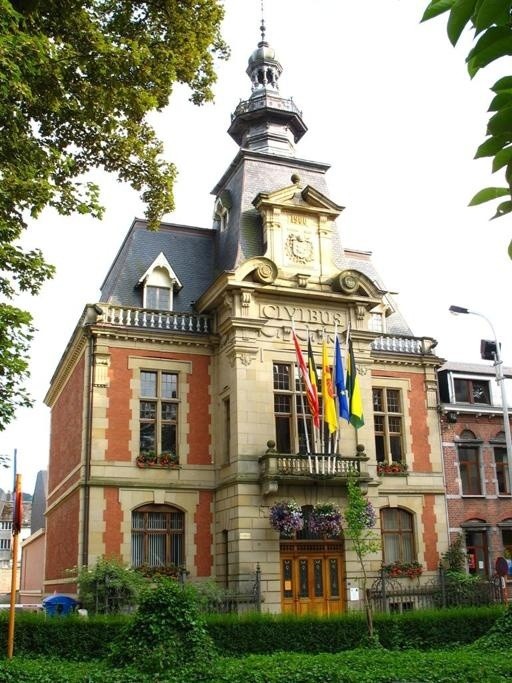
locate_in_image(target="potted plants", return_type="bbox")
[160,449,176,464]
[143,450,156,465]
[377,462,408,472]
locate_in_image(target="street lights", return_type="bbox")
[448,305,511,489]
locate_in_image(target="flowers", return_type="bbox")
[307,502,344,536]
[383,559,424,578]
[269,497,304,536]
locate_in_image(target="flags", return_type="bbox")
[345,325,365,429]
[321,326,339,435]
[333,322,351,424]
[291,316,317,417]
[305,327,321,431]
